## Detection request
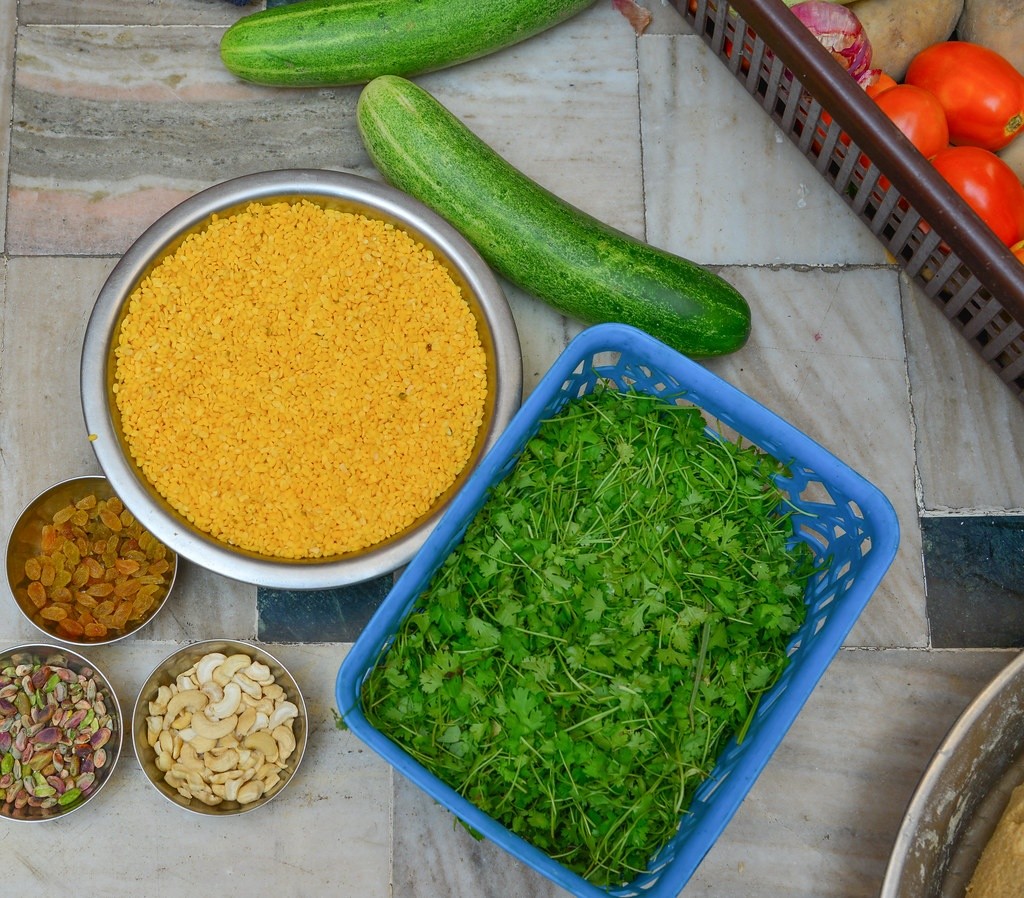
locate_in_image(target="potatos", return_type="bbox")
[846,0,1024,186]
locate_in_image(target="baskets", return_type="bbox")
[335,323,902,898]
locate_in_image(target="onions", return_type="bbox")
[763,0,874,105]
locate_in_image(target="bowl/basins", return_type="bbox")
[130,637,310,818]
[81,167,526,591]
[3,475,181,647]
[872,641,1023,896]
[0,643,124,825]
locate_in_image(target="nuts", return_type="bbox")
[24,495,169,636]
[1,661,113,810]
[145,653,299,804]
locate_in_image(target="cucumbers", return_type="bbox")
[217,0,601,89]
[354,75,753,361]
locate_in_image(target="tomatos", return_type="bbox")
[799,42,1024,266]
[725,26,766,71]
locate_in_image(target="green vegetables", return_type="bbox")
[330,366,833,894]
